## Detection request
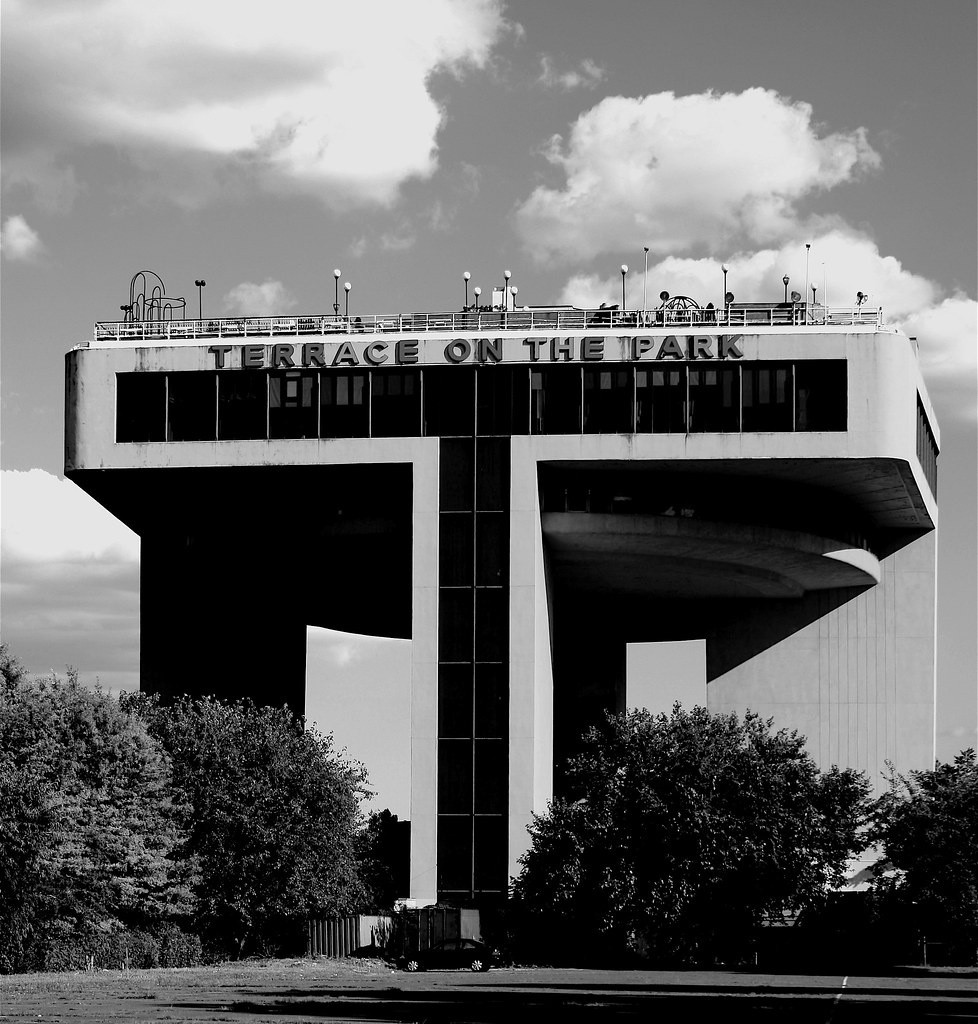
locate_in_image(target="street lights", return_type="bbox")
[783,274,790,303]
[332,269,341,334]
[463,271,472,328]
[805,243,811,326]
[503,270,511,317]
[510,286,518,312]
[343,282,352,321]
[643,247,651,315]
[474,287,484,311]
[721,264,729,321]
[194,279,206,325]
[811,281,818,303]
[620,265,628,321]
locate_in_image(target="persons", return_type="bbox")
[298,318,315,329]
[206,322,219,332]
[629,302,716,323]
[354,317,364,333]
[436,945,441,950]
[460,303,508,312]
[461,942,467,949]
[600,303,606,309]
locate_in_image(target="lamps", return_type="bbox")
[724,292,734,303]
[792,290,802,303]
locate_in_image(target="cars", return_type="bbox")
[395,938,497,973]
